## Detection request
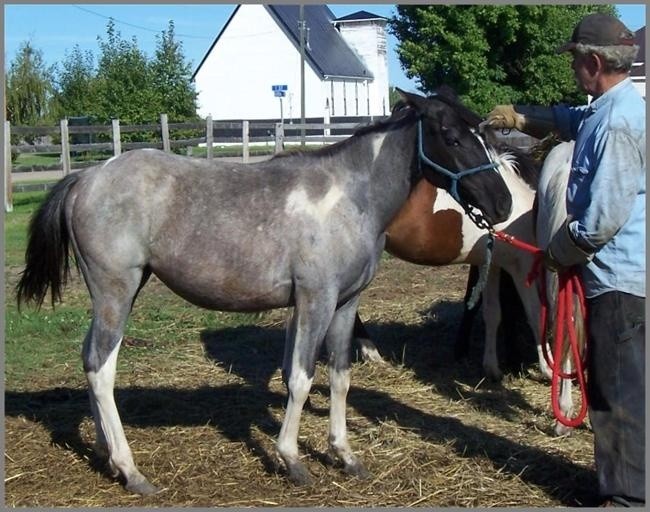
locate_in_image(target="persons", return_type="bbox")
[476,11,646,506]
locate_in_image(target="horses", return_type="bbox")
[14,86,515,493]
[354,113,578,436]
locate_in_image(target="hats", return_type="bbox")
[555,13,636,55]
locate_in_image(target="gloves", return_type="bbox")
[486,104,556,140]
[544,215,594,280]
